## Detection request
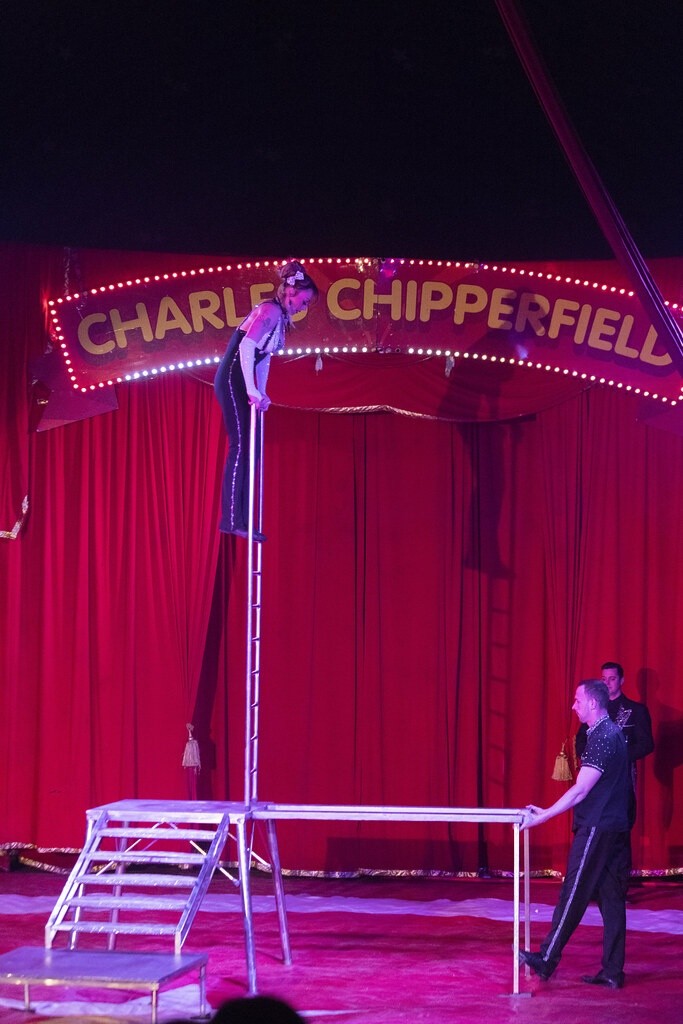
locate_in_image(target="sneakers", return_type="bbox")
[221,523,266,542]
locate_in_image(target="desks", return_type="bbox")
[0,945,207,1024]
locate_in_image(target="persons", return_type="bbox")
[213,261,320,543]
[513,661,654,988]
[162,996,309,1024]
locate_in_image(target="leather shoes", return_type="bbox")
[581,970,623,990]
[520,949,556,980]
